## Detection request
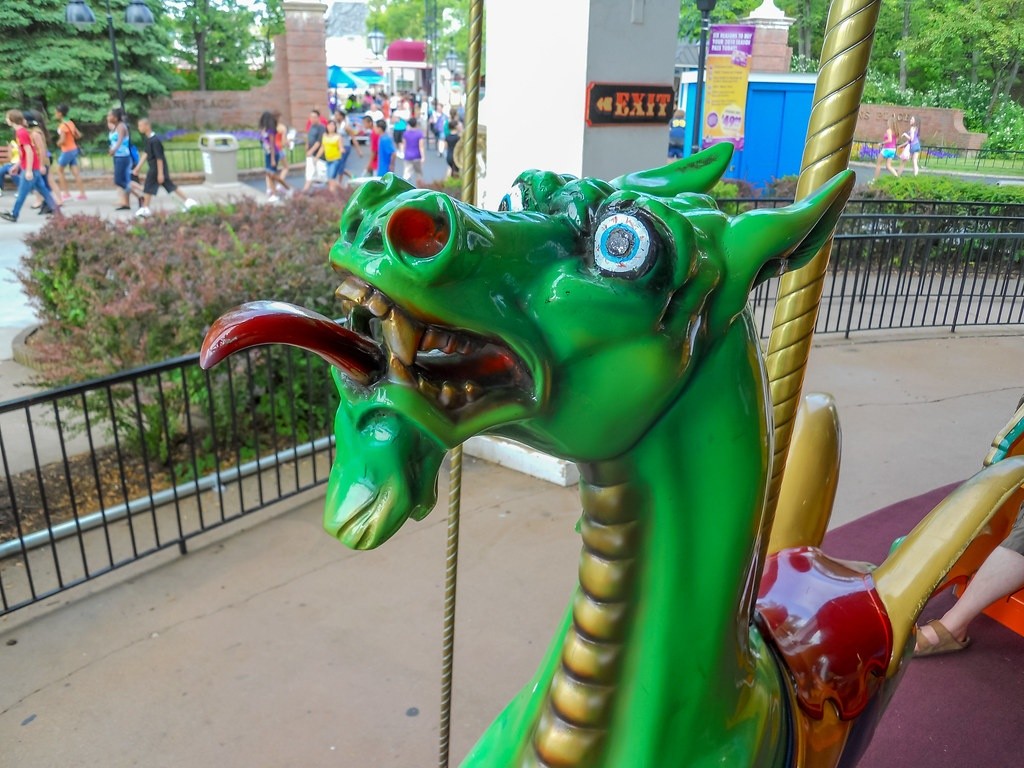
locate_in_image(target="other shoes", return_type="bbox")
[30,201,41,208]
[61,192,72,201]
[116,206,130,211]
[269,194,280,202]
[136,208,152,217]
[0,212,16,222]
[75,194,86,200]
[285,187,293,198]
[139,197,144,206]
[182,197,198,212]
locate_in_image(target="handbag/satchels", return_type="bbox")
[899,143,910,160]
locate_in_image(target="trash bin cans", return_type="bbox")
[199,134,240,189]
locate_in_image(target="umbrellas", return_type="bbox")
[347,67,385,87]
[324,64,358,107]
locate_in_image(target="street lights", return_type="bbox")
[65,0,156,182]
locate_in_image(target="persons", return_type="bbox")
[429,102,464,178]
[0,109,64,222]
[867,116,901,186]
[398,117,427,184]
[257,110,294,204]
[313,121,346,190]
[104,108,147,210]
[372,118,396,176]
[331,110,363,183]
[911,494,1024,657]
[667,109,686,163]
[132,115,197,217]
[53,102,88,201]
[302,110,329,195]
[360,115,380,175]
[894,114,922,178]
[327,88,433,145]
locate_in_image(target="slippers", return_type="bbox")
[913,619,970,655]
